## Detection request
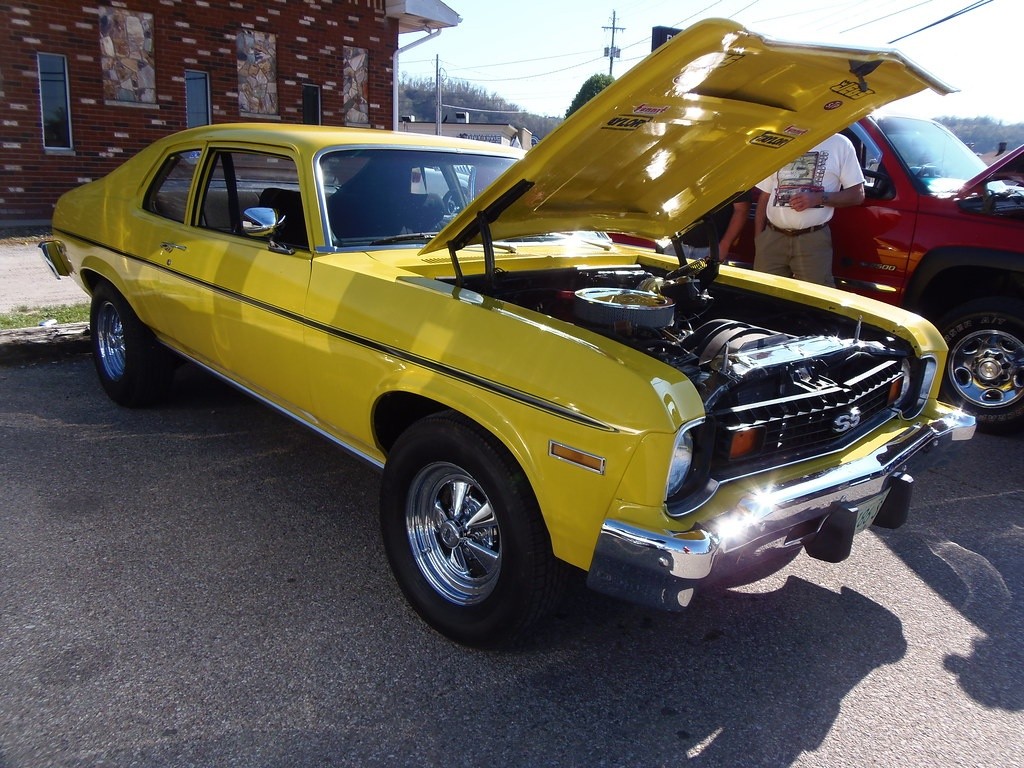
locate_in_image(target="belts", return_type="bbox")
[765,217,827,235]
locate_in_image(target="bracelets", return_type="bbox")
[822,191,830,203]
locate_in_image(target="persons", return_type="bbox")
[753,133,865,290]
[663,190,752,264]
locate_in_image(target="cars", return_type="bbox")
[36,14,979,652]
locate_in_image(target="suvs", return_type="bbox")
[600,111,1024,440]
